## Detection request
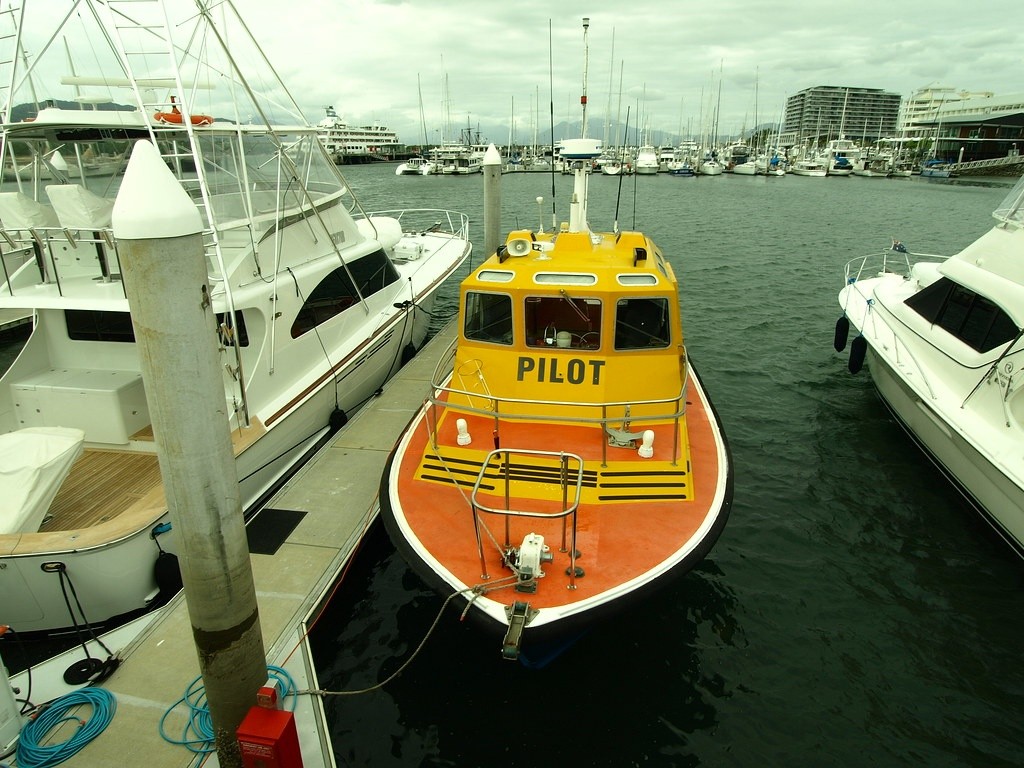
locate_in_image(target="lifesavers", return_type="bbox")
[153,112,214,127]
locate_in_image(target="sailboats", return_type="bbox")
[393,67,948,178]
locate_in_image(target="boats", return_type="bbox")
[1,0,473,646]
[380,163,735,661]
[831,173,1024,571]
[0,180,66,337]
